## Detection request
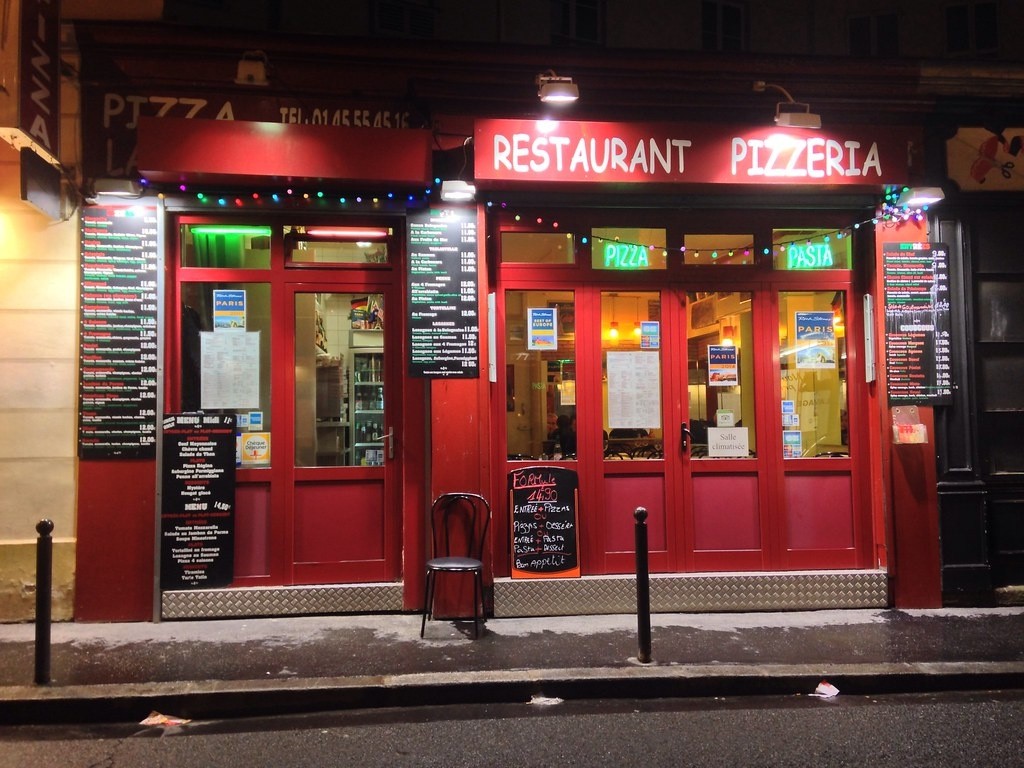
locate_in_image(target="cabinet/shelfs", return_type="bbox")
[347,346,384,465]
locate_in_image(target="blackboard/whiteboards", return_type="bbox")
[508,466,582,579]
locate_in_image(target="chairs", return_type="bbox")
[421,492,492,641]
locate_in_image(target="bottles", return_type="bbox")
[356,385,383,410]
[356,420,384,442]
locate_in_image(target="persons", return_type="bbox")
[602,428,650,456]
[547,413,577,461]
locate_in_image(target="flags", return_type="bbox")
[351,296,368,313]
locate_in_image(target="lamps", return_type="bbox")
[608,293,619,332]
[233,49,270,88]
[633,298,641,329]
[440,181,483,202]
[752,81,821,129]
[537,69,579,104]
[896,186,945,207]
[93,177,140,196]
[722,326,734,340]
